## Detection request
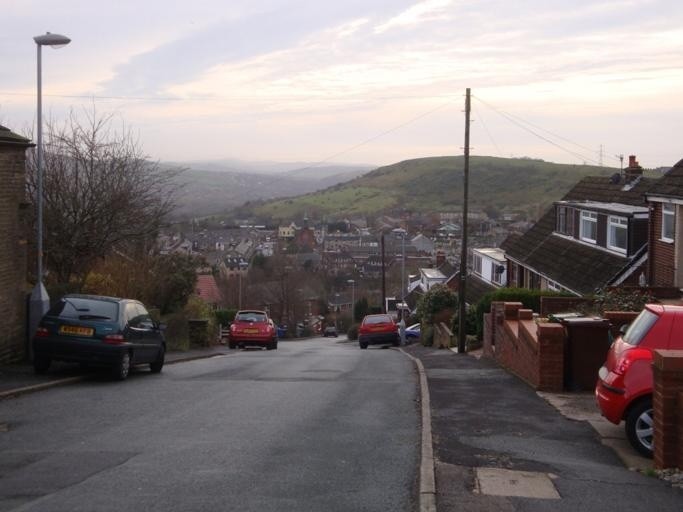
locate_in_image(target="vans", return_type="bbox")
[32,292,167,381]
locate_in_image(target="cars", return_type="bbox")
[406,323,421,343]
[275,325,288,337]
[357,313,401,349]
[324,326,338,338]
[595,302,683,458]
[229,311,276,350]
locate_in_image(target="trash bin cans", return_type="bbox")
[296,324,305,338]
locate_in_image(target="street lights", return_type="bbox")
[32,30,72,287]
[392,227,408,320]
[347,279,355,323]
[238,262,249,310]
